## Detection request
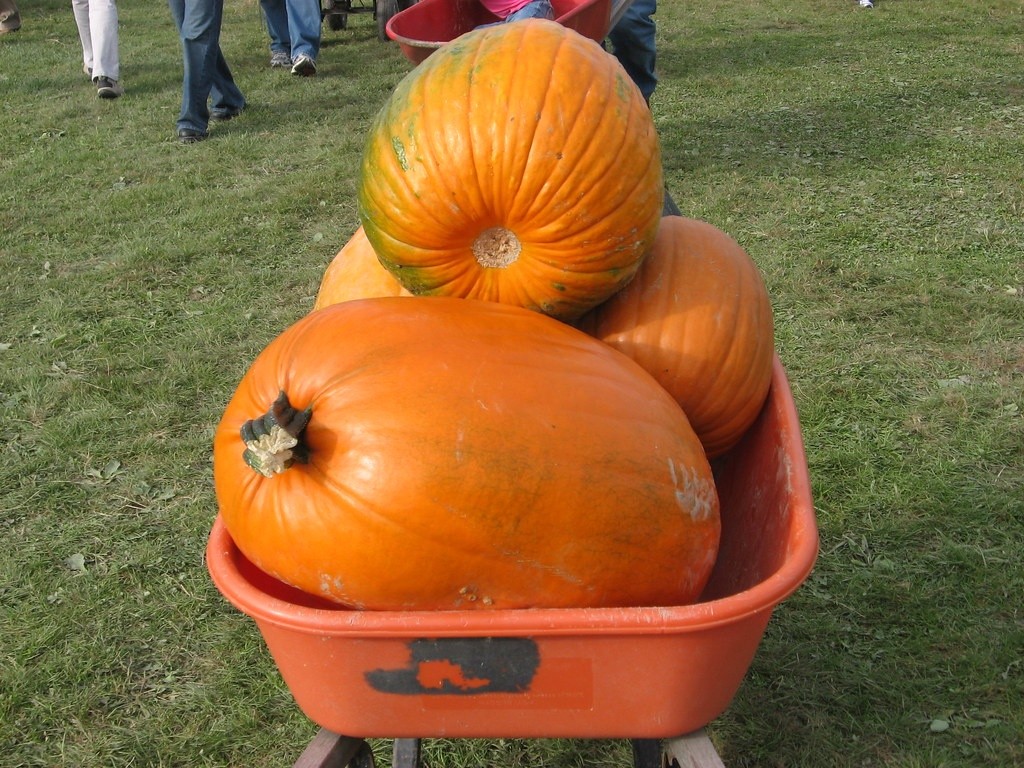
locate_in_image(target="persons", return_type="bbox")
[169,1,248,144]
[0,0,22,33]
[72,0,120,98]
[258,0,322,75]
[470,0,555,32]
[609,0,657,109]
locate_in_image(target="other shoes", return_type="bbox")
[178,128,206,142]
[83,65,93,75]
[210,112,238,121]
[860,0,873,9]
[96,75,121,98]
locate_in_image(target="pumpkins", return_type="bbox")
[210,296,722,611]
[578,215,775,457]
[313,225,410,309]
[358,17,665,322]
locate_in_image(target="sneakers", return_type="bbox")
[270,52,292,67]
[291,52,316,77]
